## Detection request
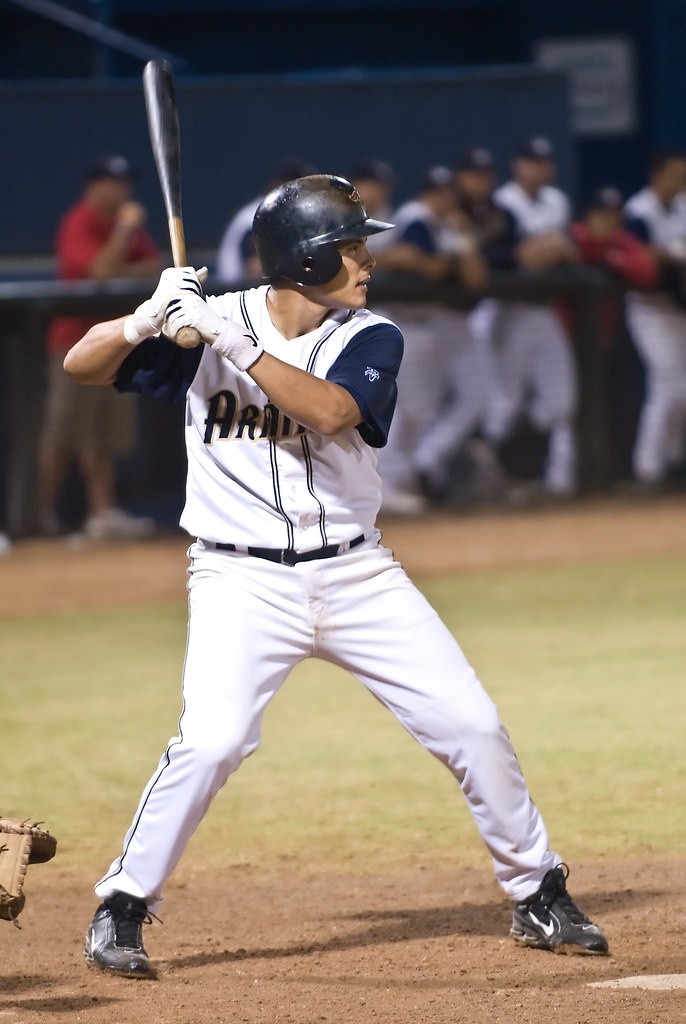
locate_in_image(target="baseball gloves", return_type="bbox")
[0,816,58,929]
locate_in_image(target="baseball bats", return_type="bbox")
[142,59,202,350]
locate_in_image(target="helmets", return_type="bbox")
[252,174,395,285]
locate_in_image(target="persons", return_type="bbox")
[63,174,611,979]
[219,141,685,513]
[37,156,164,540]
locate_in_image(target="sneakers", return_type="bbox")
[83,889,164,980]
[510,863,608,955]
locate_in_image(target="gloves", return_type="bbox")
[162,294,230,346]
[140,266,208,331]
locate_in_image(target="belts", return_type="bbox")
[216,534,364,567]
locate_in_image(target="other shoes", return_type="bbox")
[85,508,156,543]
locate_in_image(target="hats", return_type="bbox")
[336,135,633,215]
[83,154,134,180]
